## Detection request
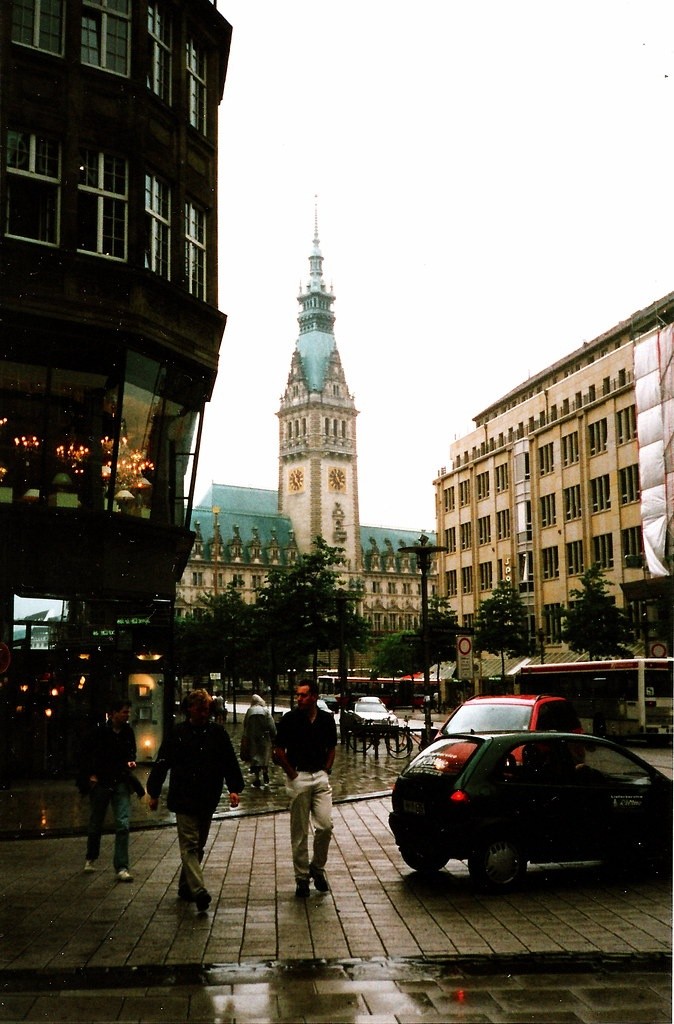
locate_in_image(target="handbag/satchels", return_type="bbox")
[239,735,250,762]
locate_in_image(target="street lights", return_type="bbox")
[533,627,552,664]
[397,535,450,749]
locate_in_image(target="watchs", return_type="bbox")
[323,768,332,774]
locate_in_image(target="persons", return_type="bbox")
[146,688,245,912]
[77,698,145,882]
[240,694,277,786]
[273,678,337,898]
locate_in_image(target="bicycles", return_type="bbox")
[386,714,425,753]
[348,717,413,760]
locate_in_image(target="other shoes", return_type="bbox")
[195,890,212,913]
[252,779,262,788]
[262,770,269,784]
[177,889,197,902]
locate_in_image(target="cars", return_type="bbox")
[321,696,339,713]
[317,700,334,718]
[388,729,674,891]
[430,694,588,770]
[341,702,398,729]
[358,697,386,708]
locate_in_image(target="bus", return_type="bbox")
[286,668,403,696]
[513,657,674,746]
[316,675,501,707]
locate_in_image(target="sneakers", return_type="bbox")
[308,863,329,892]
[84,860,97,873]
[294,880,311,898]
[114,868,134,883]
